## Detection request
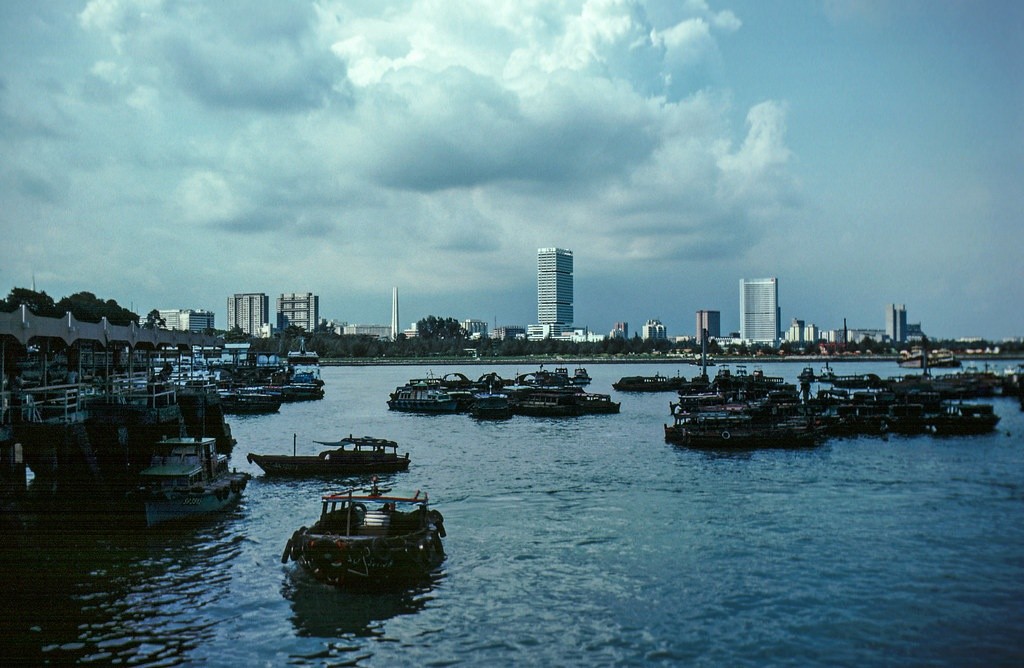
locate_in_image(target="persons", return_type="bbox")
[147,364,173,408]
[7,370,32,390]
[66,364,79,385]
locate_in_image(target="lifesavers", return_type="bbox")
[721,431,730,439]
[281,525,306,564]
[232,474,251,493]
[215,486,230,501]
[405,509,446,564]
[350,504,367,521]
[315,541,338,564]
[371,537,392,562]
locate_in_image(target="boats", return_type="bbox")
[611,373,676,392]
[0,335,327,536]
[247,432,412,482]
[386,362,621,422]
[665,332,1023,454]
[280,474,446,589]
